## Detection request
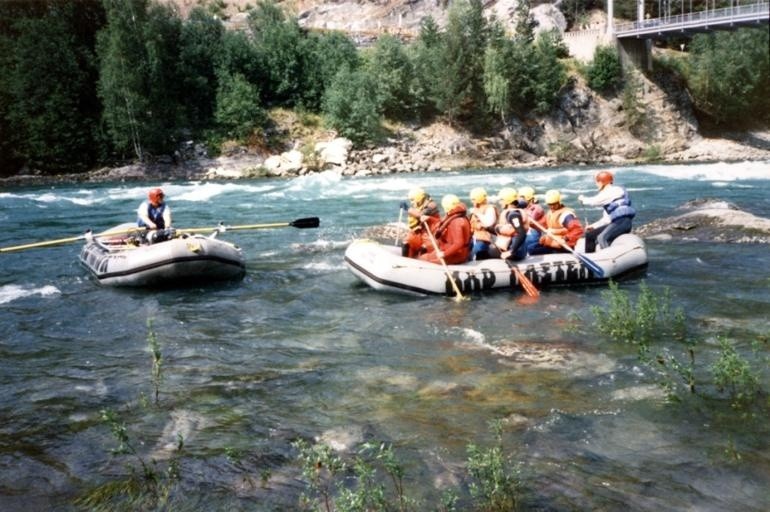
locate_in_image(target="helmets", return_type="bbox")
[441,194,460,212]
[498,186,535,207]
[596,171,613,184]
[149,189,165,204]
[544,190,562,206]
[469,187,486,206]
[409,188,426,206]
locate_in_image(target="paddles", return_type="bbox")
[482,227,540,298]
[529,219,605,278]
[176,217,319,233]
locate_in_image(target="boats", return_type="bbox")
[344,232,649,298]
[78,222,247,289]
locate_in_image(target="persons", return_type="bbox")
[420,194,472,266]
[490,188,528,261]
[578,172,634,253]
[137,188,173,244]
[466,187,499,262]
[518,185,545,254]
[403,189,438,260]
[531,189,582,252]
